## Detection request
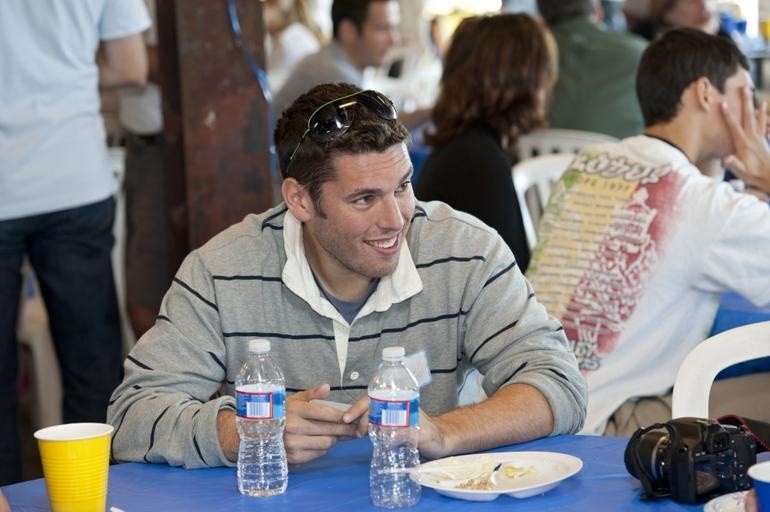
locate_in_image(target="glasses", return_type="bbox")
[285,89,399,179]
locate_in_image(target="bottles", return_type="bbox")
[235,338,291,499]
[367,346,423,508]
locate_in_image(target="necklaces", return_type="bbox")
[644,133,692,162]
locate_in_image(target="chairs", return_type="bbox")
[511,152,582,261]
[515,125,629,213]
[666,319,770,420]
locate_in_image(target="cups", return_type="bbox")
[34,422,115,512]
[745,459,770,512]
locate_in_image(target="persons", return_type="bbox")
[263,0,473,130]
[104,83,589,470]
[419,1,724,271]
[457,27,770,434]
[0,0,156,486]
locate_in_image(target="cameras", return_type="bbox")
[624,417,757,505]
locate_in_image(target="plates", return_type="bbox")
[704,490,746,511]
[406,451,585,503]
